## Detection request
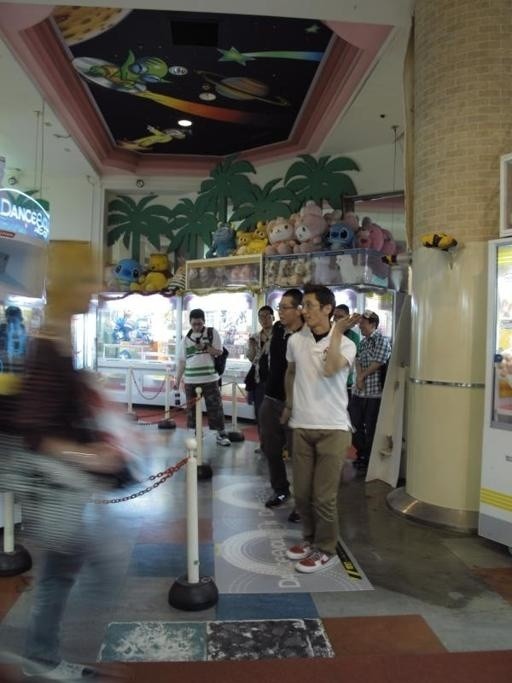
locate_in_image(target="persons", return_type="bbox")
[2,303,31,369]
[244,285,393,523]
[273,285,364,574]
[3,239,149,681]
[172,308,232,446]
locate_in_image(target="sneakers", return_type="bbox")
[288,509,301,522]
[216,435,231,446]
[265,489,292,508]
[286,540,315,560]
[295,547,339,573]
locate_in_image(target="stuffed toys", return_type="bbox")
[111,201,397,296]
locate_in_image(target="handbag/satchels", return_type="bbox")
[214,347,228,374]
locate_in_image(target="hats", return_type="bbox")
[362,309,378,321]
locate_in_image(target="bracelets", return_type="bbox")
[202,343,208,350]
